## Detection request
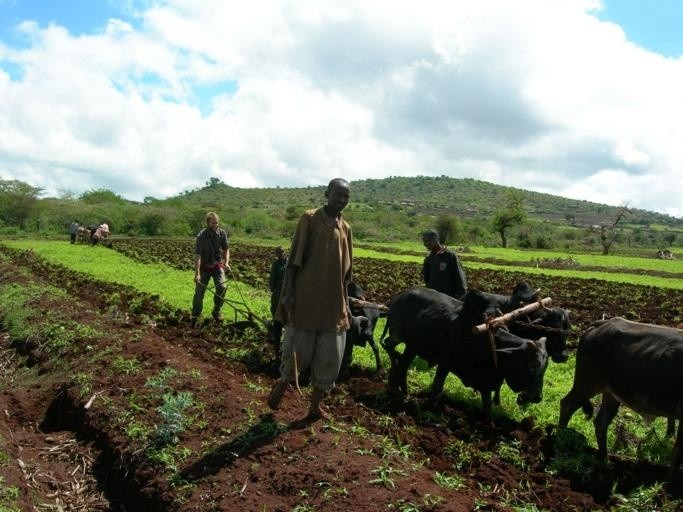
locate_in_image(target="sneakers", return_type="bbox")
[308,406,332,422]
[268,377,289,411]
[212,310,224,323]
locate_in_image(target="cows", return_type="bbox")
[430,288,571,406]
[559,317,683,469]
[82,226,103,246]
[379,288,548,418]
[270,280,389,374]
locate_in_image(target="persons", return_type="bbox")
[268,176,353,423]
[656,249,675,260]
[191,210,232,322]
[421,228,468,300]
[269,244,289,317]
[68,217,110,247]
